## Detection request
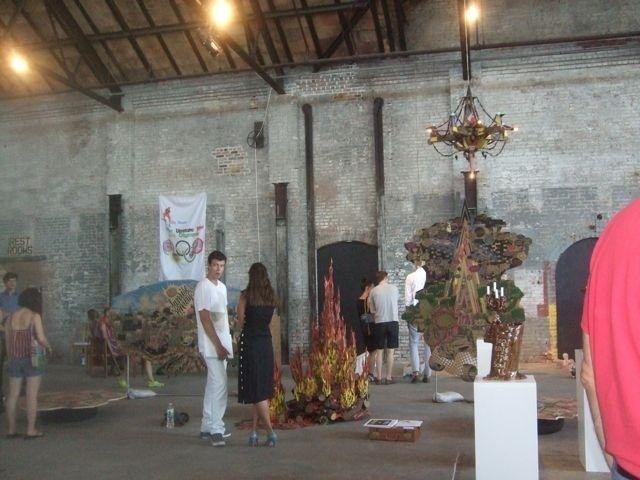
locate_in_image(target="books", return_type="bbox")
[363,418,399,428]
[395,419,423,428]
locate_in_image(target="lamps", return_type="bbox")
[426,0,517,185]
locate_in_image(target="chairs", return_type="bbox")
[89,336,131,380]
[70,326,97,362]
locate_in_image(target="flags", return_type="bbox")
[157,191,207,281]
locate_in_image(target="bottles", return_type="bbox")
[165,402,175,429]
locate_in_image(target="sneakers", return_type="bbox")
[423,377,430,383]
[374,377,382,385]
[118,379,130,388]
[411,375,420,383]
[210,433,226,446]
[386,377,395,385]
[148,381,164,387]
[200,431,232,439]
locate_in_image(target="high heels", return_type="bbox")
[263,432,277,447]
[249,431,257,448]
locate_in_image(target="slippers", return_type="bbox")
[25,431,45,438]
[7,430,23,437]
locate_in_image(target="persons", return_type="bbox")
[578,196,640,478]
[0,272,22,402]
[3,287,53,439]
[358,278,377,383]
[192,250,234,446]
[96,304,166,388]
[365,270,400,387]
[231,262,280,447]
[404,260,433,383]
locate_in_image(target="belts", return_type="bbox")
[616,463,639,479]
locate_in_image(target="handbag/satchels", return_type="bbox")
[360,313,374,323]
[30,340,51,368]
